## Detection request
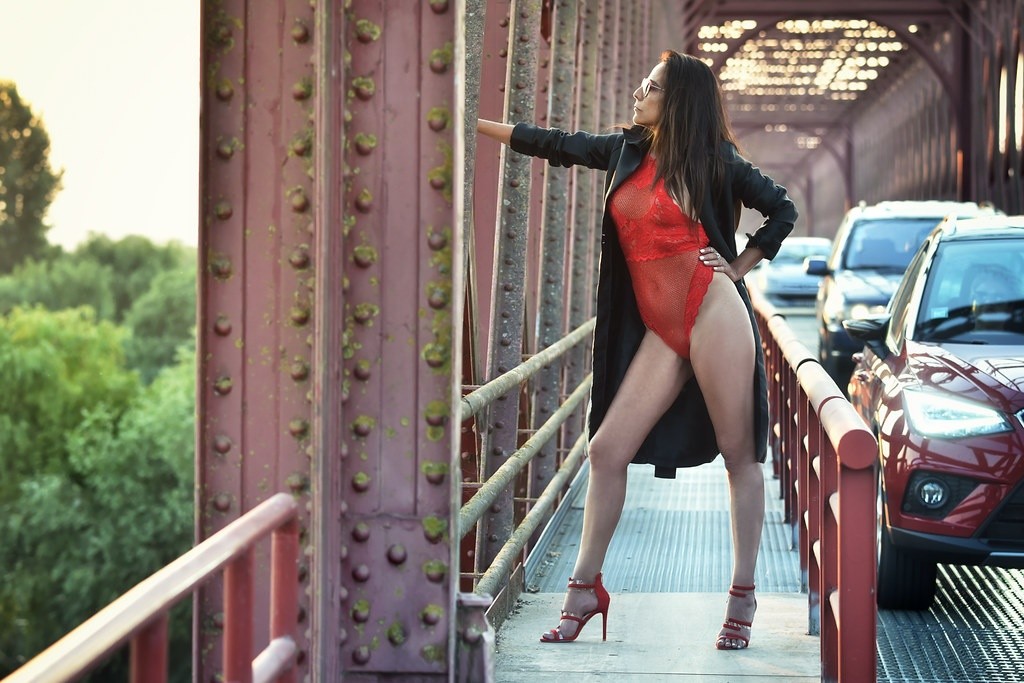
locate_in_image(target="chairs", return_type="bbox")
[852,238,898,266]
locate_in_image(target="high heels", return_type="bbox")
[717,585,757,651]
[541,573,611,643]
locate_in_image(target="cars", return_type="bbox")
[735,233,761,269]
[761,237,833,309]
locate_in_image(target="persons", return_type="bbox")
[970,272,1015,323]
[476,50,799,649]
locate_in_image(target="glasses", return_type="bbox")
[641,77,665,96]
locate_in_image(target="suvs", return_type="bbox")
[840,216,1024,613]
[802,199,1010,391]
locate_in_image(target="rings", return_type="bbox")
[715,253,720,259]
